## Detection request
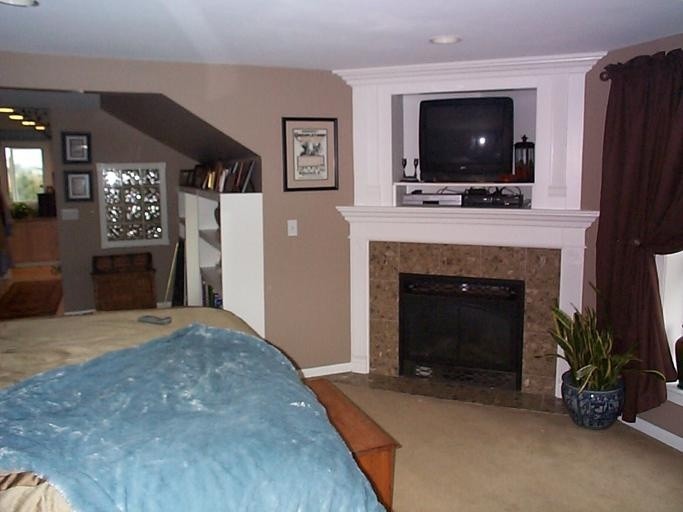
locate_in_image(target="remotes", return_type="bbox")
[138,315,171,324]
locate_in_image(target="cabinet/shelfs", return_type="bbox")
[92,252,157,311]
[175,185,265,342]
[11,221,62,267]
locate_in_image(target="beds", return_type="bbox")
[1,303,394,511]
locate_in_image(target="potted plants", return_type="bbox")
[537,280,667,430]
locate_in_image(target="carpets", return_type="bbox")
[0,281,63,320]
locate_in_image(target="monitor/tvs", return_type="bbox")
[419,97,514,183]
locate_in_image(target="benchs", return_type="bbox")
[302,376,398,512]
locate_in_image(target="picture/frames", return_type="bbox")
[61,131,91,163]
[282,117,339,192]
[178,160,257,193]
[63,171,93,203]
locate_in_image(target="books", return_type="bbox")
[202,282,221,308]
